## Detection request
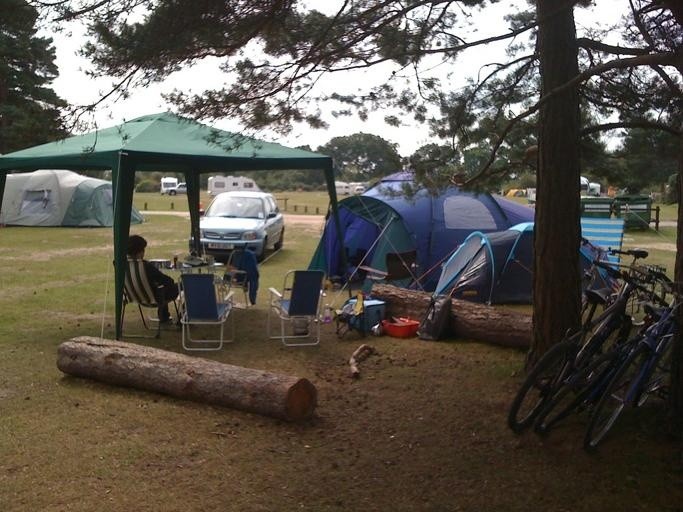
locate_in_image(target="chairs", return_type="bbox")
[358,249,416,289]
[113,248,257,352]
[267,270,327,347]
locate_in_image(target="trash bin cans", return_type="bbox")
[579,196,613,218]
[614,194,652,231]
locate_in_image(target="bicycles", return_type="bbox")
[507,232,681,454]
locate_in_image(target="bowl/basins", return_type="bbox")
[381,317,420,337]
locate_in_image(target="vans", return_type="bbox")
[205,176,264,195]
[158,177,178,195]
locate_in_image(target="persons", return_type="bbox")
[126,234,183,326]
[621,187,630,196]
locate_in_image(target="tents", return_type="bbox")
[0,170,146,227]
[504,188,527,198]
[433,221,623,305]
[308,159,536,294]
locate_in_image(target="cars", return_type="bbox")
[167,181,188,196]
[334,181,367,195]
[199,191,285,267]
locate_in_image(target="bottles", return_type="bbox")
[174,255,178,268]
[324,304,331,324]
